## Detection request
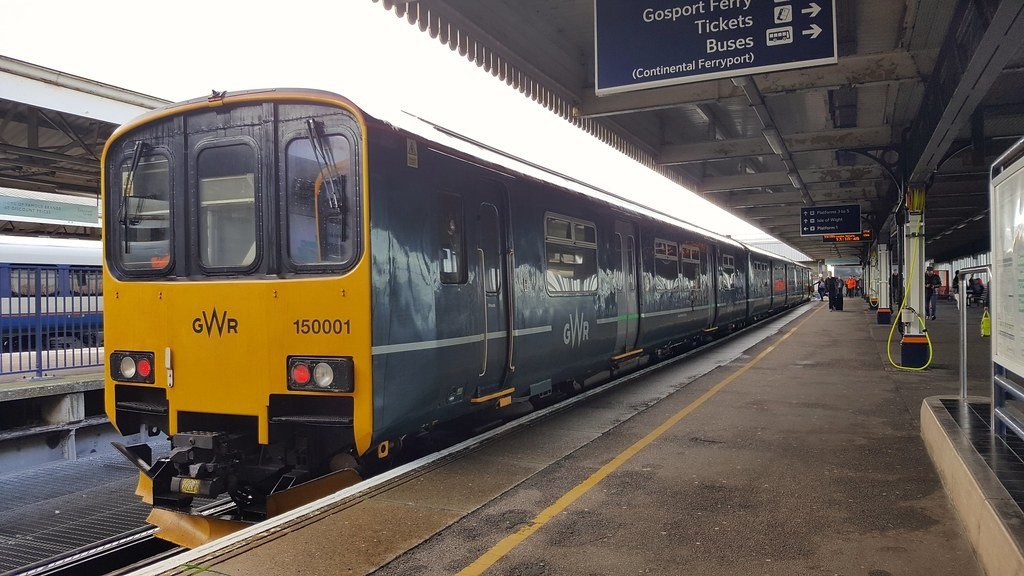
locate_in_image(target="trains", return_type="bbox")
[0,235,169,353]
[99,88,815,550]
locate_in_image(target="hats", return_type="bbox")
[926,266,934,271]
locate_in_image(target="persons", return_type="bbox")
[846,274,864,298]
[984,282,989,311]
[824,271,837,310]
[953,269,959,310]
[925,266,941,320]
[889,269,899,303]
[814,276,828,301]
[838,276,844,294]
[967,278,983,306]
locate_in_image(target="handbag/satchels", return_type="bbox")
[819,281,826,288]
[980,311,991,337]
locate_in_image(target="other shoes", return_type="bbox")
[931,315,936,320]
[829,308,832,312]
[926,315,929,319]
